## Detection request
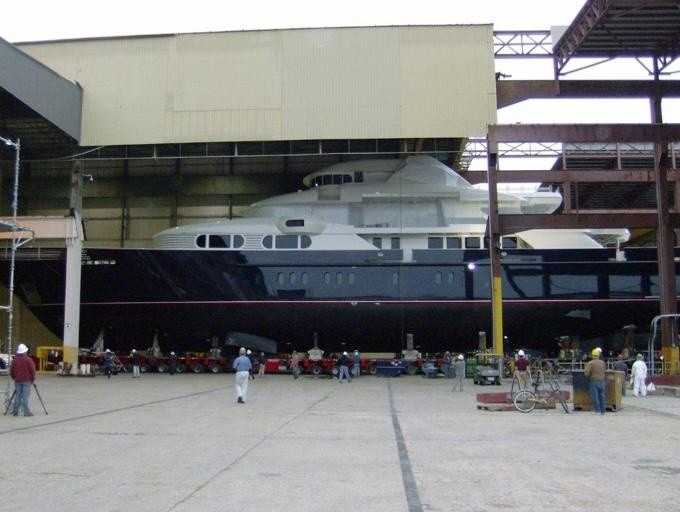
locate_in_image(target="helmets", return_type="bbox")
[342,351,348,355]
[132,349,137,353]
[616,354,624,360]
[247,349,252,354]
[591,346,602,357]
[16,343,29,354]
[458,354,464,360]
[105,349,111,352]
[239,347,246,353]
[518,350,524,356]
[170,351,175,355]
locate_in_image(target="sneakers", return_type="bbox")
[237,397,246,403]
[596,411,607,415]
[13,412,18,416]
[24,412,34,417]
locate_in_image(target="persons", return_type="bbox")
[443,351,451,379]
[353,350,361,378]
[79,348,112,378]
[451,354,466,392]
[334,351,352,383]
[130,349,140,379]
[169,352,177,375]
[290,350,298,379]
[585,346,648,415]
[515,349,531,392]
[232,346,266,402]
[10,344,35,416]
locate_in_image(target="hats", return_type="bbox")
[635,353,644,359]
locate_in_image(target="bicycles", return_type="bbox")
[512,373,571,413]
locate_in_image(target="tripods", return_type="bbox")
[3,384,48,416]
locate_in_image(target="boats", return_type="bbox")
[0,157,680,359]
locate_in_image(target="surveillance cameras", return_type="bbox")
[89,178,93,182]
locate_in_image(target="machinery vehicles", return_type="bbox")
[30,343,469,378]
